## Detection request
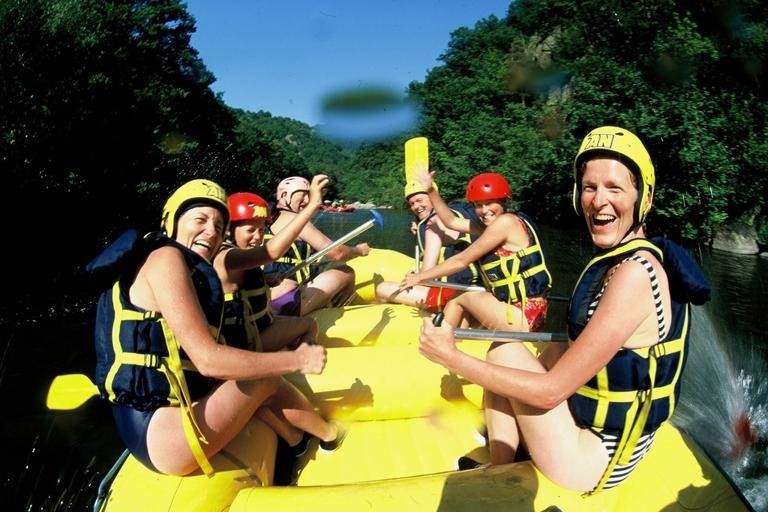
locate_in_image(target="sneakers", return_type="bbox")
[456,420,488,471]
[289,418,347,457]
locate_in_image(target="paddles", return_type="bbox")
[404,137,429,275]
[46,373,102,411]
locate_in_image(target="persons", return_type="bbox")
[375,177,481,314]
[261,175,370,317]
[398,171,549,333]
[212,172,334,353]
[87,177,350,477]
[417,125,691,497]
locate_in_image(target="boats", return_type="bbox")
[90,242,761,512]
[317,204,357,212]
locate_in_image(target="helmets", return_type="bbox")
[572,126,655,224]
[160,177,309,239]
[404,180,438,203]
[466,172,512,204]
